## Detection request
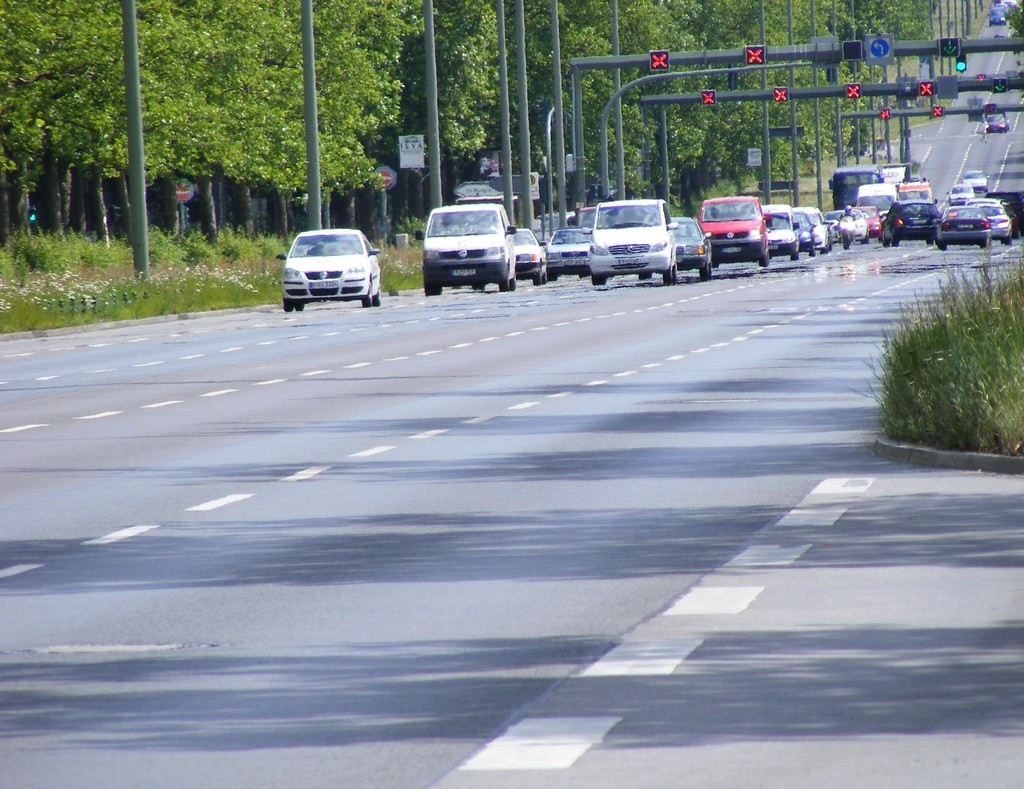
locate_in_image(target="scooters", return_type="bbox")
[837,216,856,251]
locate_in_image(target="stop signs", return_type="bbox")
[371,165,395,190]
[174,178,195,205]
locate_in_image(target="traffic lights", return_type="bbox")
[26,207,40,231]
[955,54,968,74]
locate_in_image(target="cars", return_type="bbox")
[515,162,1013,288]
[984,112,1009,133]
[988,1,1018,39]
[672,217,712,279]
[937,206,992,248]
[273,228,382,312]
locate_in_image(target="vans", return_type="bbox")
[697,196,771,268]
[413,203,517,296]
[581,200,680,285]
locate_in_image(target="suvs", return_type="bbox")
[879,200,941,246]
